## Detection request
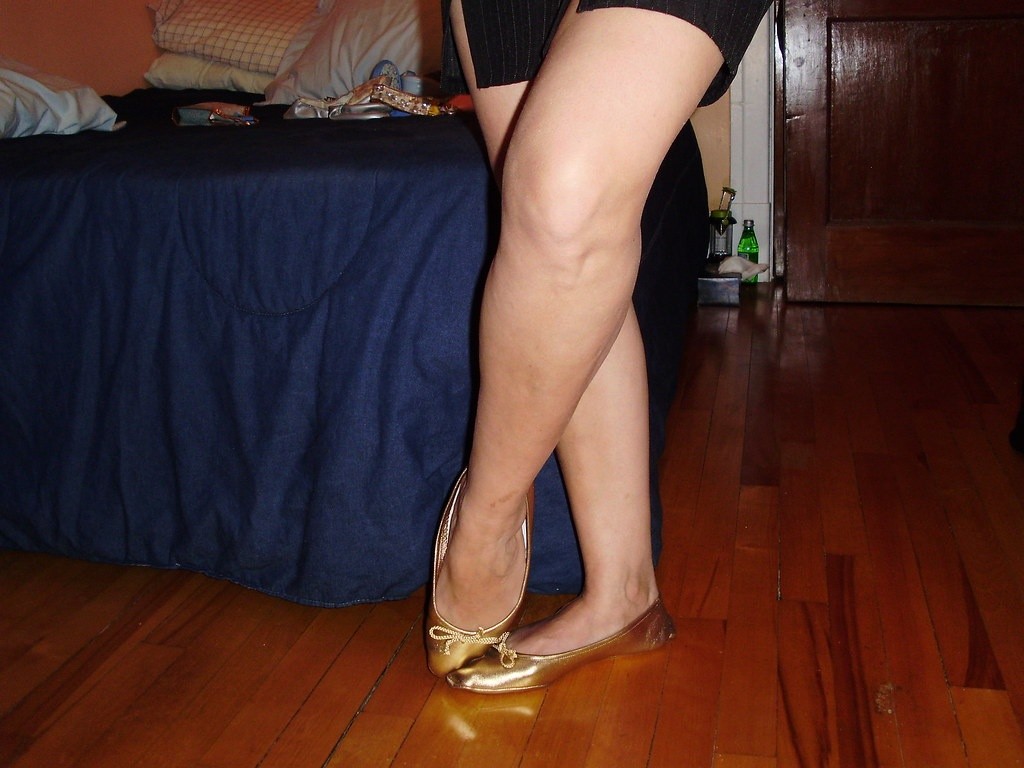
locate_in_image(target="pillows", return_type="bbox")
[144,0,442,105]
[0,52,127,139]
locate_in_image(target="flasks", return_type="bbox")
[711,208,732,263]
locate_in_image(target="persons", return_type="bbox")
[423,0,773,693]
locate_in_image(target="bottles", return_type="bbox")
[739,218,759,285]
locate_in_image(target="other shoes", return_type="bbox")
[426,465,535,677]
[445,594,676,693]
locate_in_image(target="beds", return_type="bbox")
[0,88,708,607]
